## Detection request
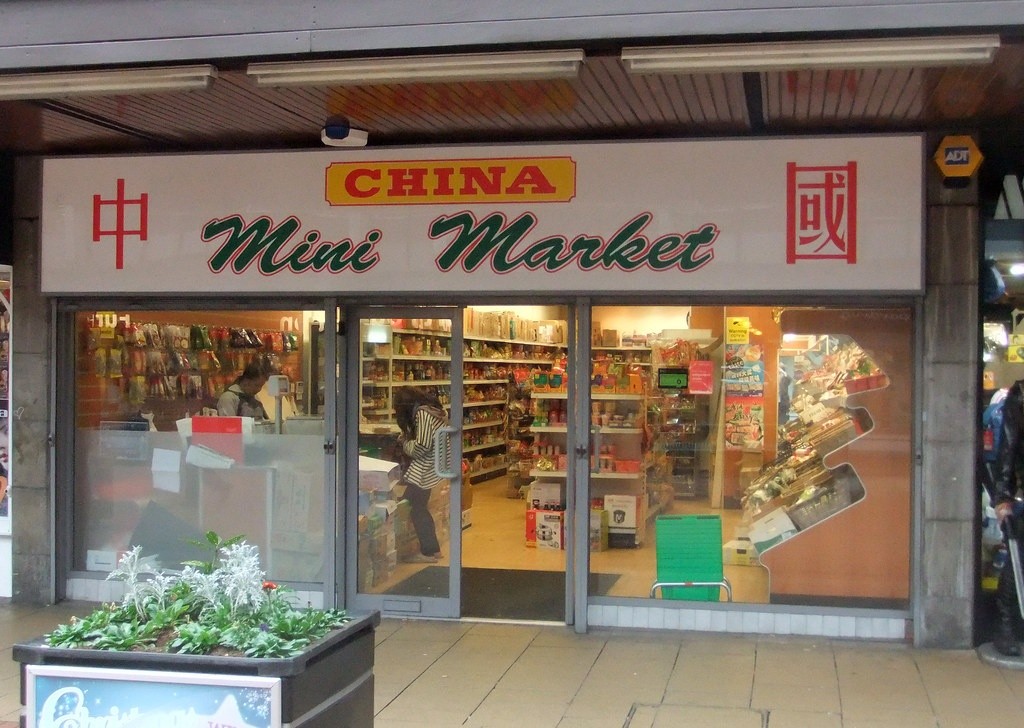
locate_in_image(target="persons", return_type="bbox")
[778,368,792,406]
[987,381,1024,655]
[391,384,451,563]
[217,364,270,424]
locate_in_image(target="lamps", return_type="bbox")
[0,63,218,101]
[247,48,587,88]
[321,128,369,147]
[621,34,1002,74]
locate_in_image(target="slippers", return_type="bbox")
[432,551,444,559]
[403,552,438,563]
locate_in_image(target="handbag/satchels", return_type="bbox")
[392,434,414,485]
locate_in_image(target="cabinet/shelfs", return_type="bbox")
[309,309,910,611]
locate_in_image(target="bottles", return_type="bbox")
[532,499,564,511]
[363,335,451,410]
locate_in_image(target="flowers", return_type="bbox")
[39,529,356,662]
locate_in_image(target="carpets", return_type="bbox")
[379,566,622,622]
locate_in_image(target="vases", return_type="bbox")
[12,610,381,728]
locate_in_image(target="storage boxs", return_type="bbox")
[603,494,641,529]
[722,540,763,567]
[563,508,609,552]
[526,509,564,550]
[530,482,566,510]
